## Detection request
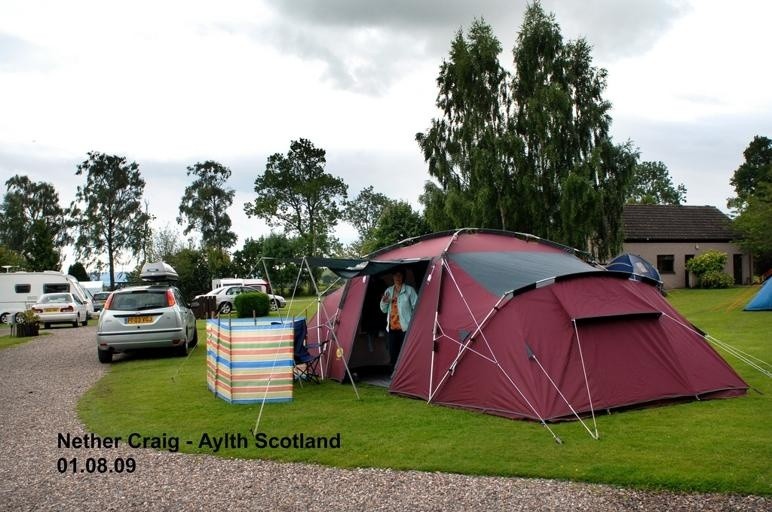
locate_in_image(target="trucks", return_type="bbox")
[212,274,266,293]
[0,264,94,324]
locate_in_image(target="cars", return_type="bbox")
[196,285,286,314]
[31,293,88,327]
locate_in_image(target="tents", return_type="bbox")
[603,253,672,297]
[169,227,772,447]
[708,268,772,312]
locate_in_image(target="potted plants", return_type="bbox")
[15,301,40,336]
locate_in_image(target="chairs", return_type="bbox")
[271,317,329,389]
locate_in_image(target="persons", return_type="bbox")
[379,271,418,376]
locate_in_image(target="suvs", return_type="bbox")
[93,292,112,312]
[96,282,197,363]
